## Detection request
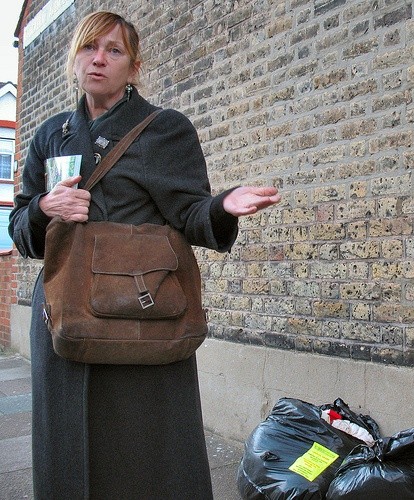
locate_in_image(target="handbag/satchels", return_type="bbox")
[41,107,209,366]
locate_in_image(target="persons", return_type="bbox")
[6,7,281,500]
